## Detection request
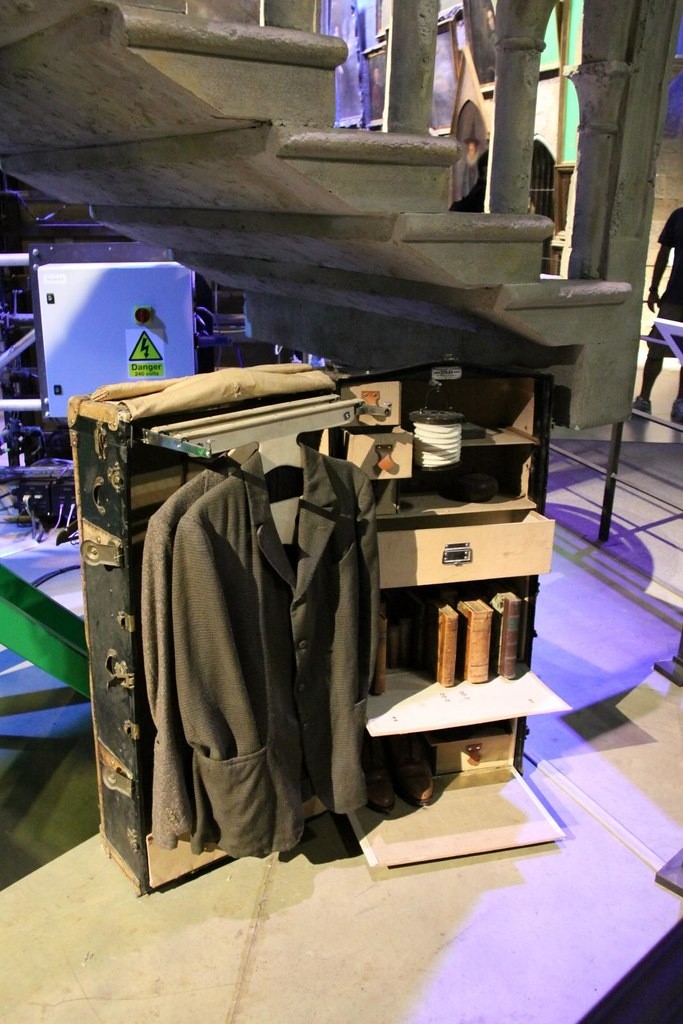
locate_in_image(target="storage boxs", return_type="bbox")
[66,359,367,897]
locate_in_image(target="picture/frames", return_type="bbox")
[320,0,497,164]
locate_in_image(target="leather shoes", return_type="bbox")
[362,731,434,813]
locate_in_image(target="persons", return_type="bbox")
[631,204,683,423]
[447,147,490,213]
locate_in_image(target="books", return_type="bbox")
[446,596,494,685]
[416,595,459,688]
[484,589,523,680]
[371,588,409,694]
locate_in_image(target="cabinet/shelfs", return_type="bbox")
[335,362,574,868]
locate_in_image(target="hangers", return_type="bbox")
[227,435,303,545]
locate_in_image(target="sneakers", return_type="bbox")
[669,396,680,422]
[633,394,652,412]
